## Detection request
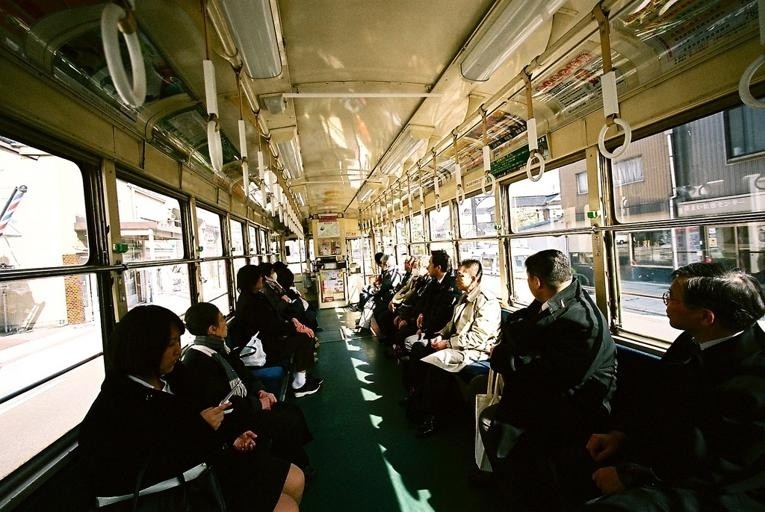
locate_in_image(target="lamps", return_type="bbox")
[212,1,309,211]
[357,0,567,204]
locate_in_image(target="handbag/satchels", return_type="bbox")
[420,347,489,372]
[475,394,502,472]
[239,330,266,366]
[93,444,227,512]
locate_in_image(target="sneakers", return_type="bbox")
[355,317,371,336]
[348,304,363,312]
[313,336,320,364]
[291,377,323,398]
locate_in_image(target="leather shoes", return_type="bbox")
[419,414,438,435]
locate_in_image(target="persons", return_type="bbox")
[77,305,306,512]
[230,260,323,397]
[579,262,765,512]
[347,250,502,438]
[183,302,319,489]
[478,249,618,512]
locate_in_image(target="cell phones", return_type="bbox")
[220,379,243,404]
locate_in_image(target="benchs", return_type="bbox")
[0,340,295,512]
[453,303,527,402]
[616,343,662,409]
